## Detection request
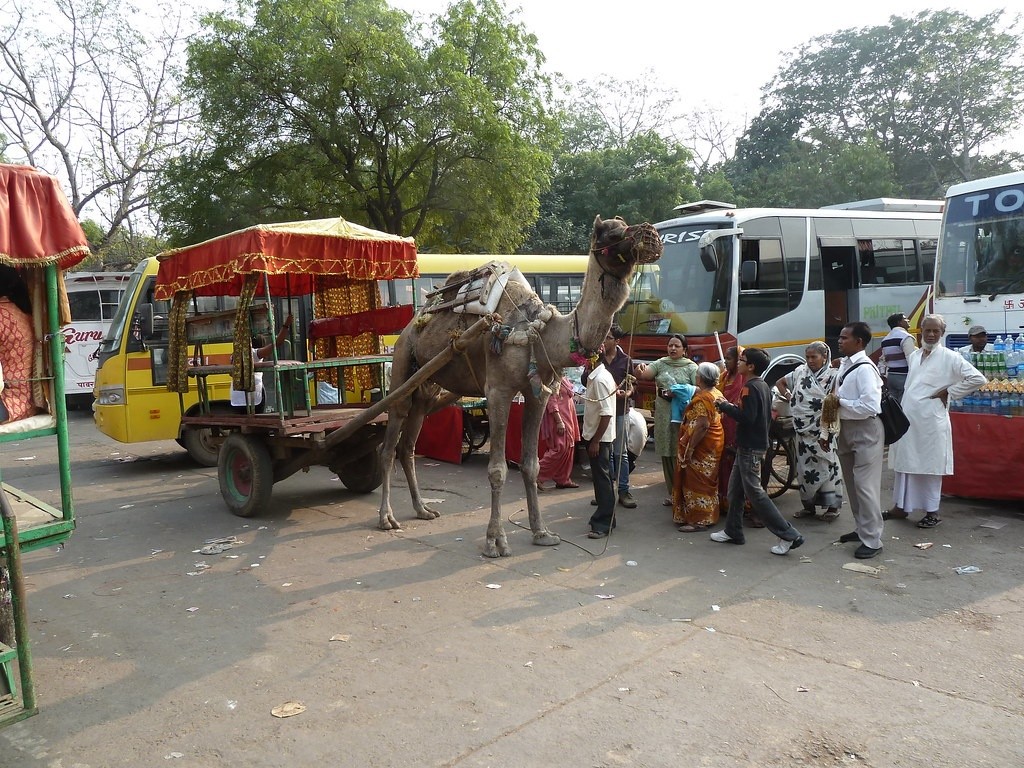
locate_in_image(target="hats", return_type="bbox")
[968,326,987,338]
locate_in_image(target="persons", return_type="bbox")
[717,347,753,517]
[878,312,921,407]
[671,361,726,533]
[774,341,846,522]
[959,325,995,360]
[818,321,886,559]
[0,263,37,425]
[230,315,295,414]
[538,371,581,491]
[882,311,988,528]
[634,331,701,508]
[621,406,648,473]
[580,322,635,506]
[710,347,806,555]
[581,344,617,540]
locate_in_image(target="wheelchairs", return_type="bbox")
[758,387,801,498]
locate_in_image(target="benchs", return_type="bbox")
[182,301,415,421]
[0,410,57,442]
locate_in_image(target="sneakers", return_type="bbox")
[710,530,745,545]
[618,492,637,507]
[771,536,804,555]
[591,499,598,505]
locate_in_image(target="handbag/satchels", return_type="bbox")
[878,391,910,445]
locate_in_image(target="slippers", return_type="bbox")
[882,510,908,520]
[916,516,942,528]
[819,511,840,521]
[793,509,817,518]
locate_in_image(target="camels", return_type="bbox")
[378,215,662,557]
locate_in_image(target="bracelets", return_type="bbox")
[555,420,562,423]
[282,324,291,329]
[782,390,791,397]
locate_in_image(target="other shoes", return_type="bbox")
[555,479,579,488]
[855,543,883,559]
[588,531,608,538]
[663,497,673,506]
[678,524,709,532]
[840,532,861,542]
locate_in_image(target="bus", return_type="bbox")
[92,254,659,466]
[612,196,966,423]
[924,171,1024,355]
[61,271,135,411]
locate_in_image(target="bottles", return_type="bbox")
[954,333,1024,382]
[950,389,1024,416]
[716,298,720,308]
[665,373,677,387]
